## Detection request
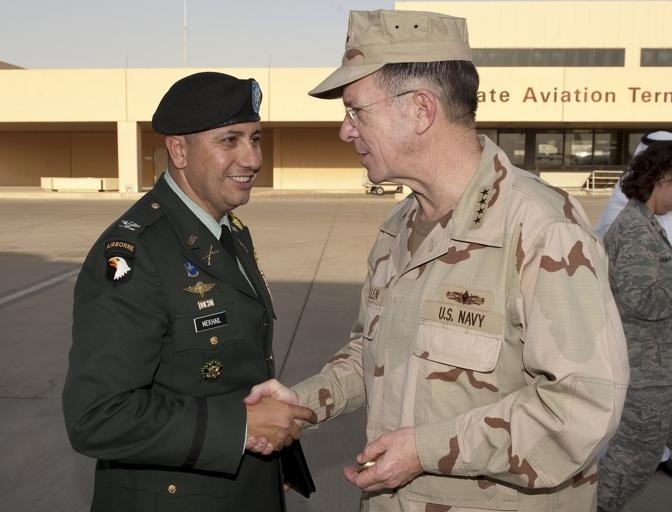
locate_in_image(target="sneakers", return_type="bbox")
[658,454,671,476]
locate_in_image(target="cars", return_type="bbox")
[364,178,403,195]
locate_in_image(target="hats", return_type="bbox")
[308,9,473,99]
[152,72,263,135]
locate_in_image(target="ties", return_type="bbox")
[219,224,237,266]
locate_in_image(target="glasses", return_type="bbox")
[344,88,440,129]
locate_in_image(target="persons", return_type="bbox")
[596,141,672,511]
[61,71,319,510]
[242,7,630,512]
[592,129,672,478]
